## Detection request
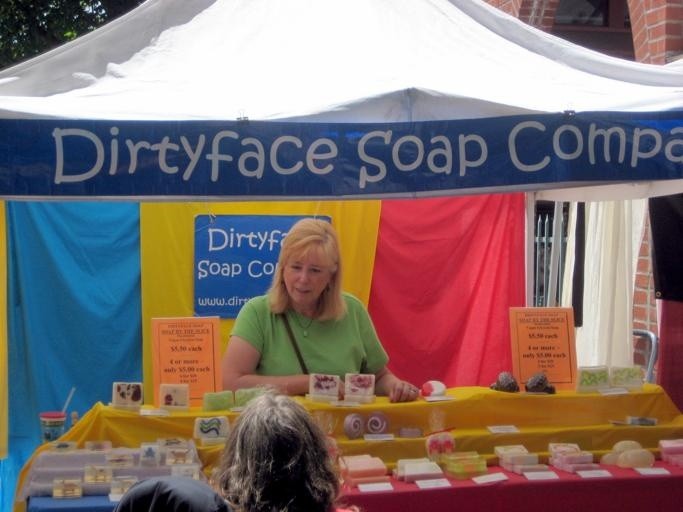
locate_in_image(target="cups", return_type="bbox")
[39,411,64,444]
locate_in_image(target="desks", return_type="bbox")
[13,383,683,512]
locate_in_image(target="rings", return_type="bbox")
[410,388,418,393]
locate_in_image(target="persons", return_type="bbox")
[219,216,420,402]
[219,390,348,512]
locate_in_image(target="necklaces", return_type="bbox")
[288,303,321,338]
